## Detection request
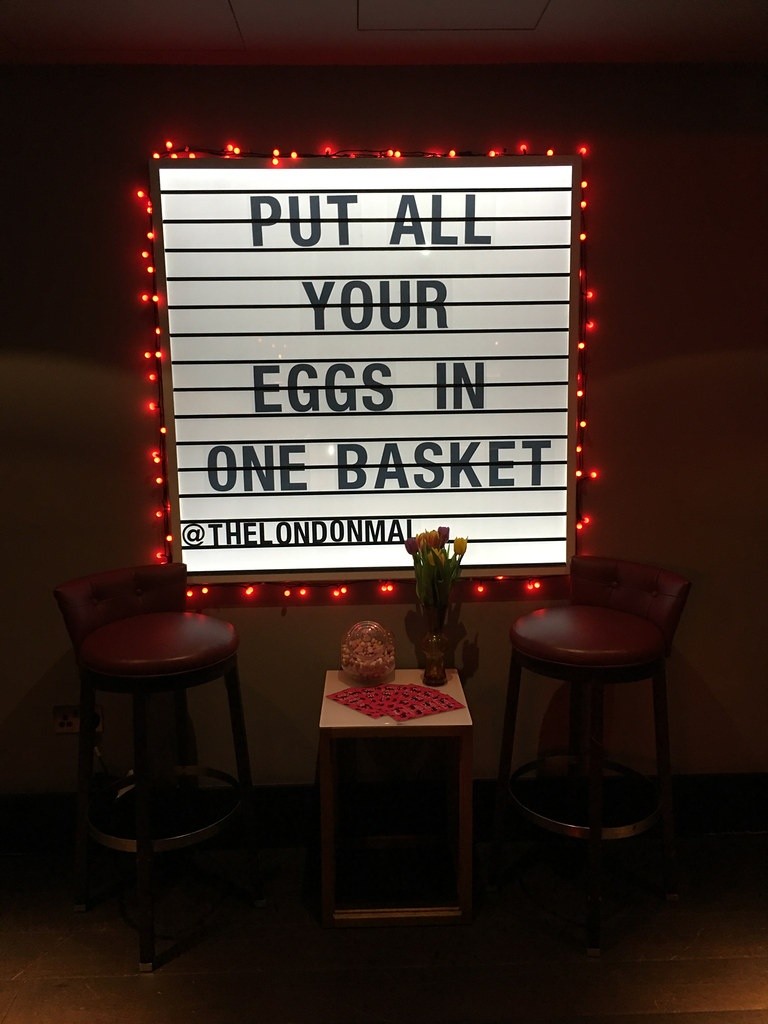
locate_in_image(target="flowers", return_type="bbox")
[404,526,467,635]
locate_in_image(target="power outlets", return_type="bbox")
[53,705,103,732]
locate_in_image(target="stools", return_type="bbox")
[485,555,691,958]
[53,563,267,970]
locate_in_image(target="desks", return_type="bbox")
[317,668,474,928]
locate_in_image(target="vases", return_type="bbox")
[416,603,449,686]
